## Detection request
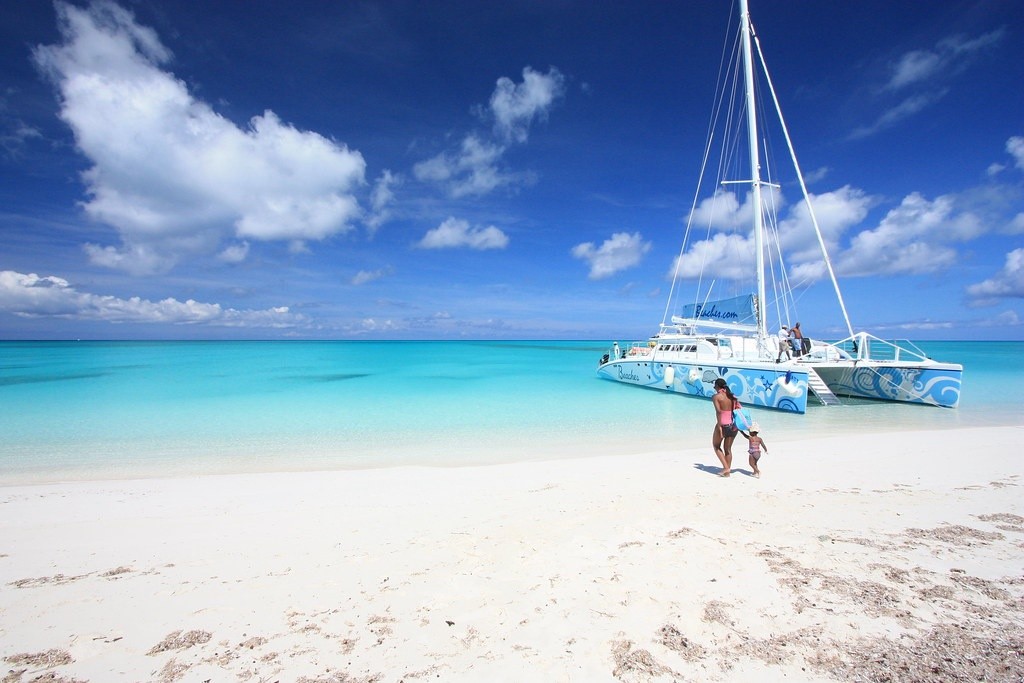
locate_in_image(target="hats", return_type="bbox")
[782,325,788,328]
[750,422,760,432]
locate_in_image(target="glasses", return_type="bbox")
[714,386,718,389]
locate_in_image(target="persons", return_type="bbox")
[777,325,792,362]
[739,423,767,476]
[788,322,805,356]
[621,350,626,358]
[614,342,620,360]
[712,378,739,477]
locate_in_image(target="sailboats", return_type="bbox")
[595,0,964,415]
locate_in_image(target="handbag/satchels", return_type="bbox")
[733,408,753,431]
